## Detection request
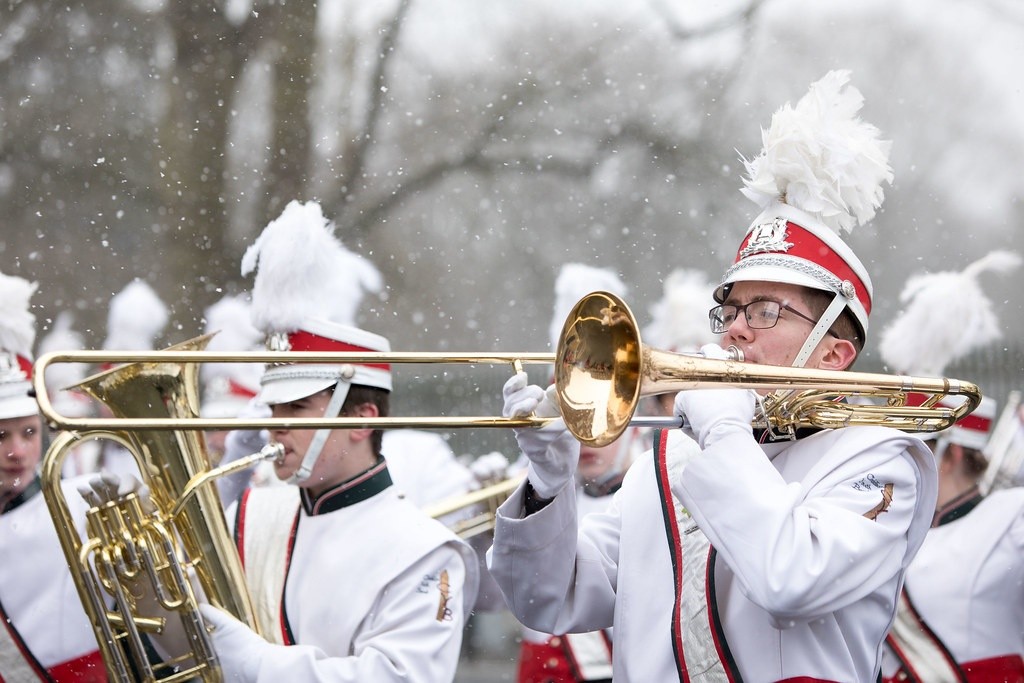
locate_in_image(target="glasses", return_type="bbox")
[708,296,841,340]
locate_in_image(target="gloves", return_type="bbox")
[672,344,756,450]
[502,372,581,498]
[198,602,267,683]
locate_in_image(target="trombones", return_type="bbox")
[29,288,983,448]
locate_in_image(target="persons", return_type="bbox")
[0,250,1024,683]
[78,199,479,683]
[487,69,938,683]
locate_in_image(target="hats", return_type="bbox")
[711,71,895,353]
[197,293,273,418]
[0,272,40,419]
[37,312,97,420]
[238,200,396,403]
[549,261,632,385]
[97,277,171,369]
[641,270,735,356]
[880,248,1024,449]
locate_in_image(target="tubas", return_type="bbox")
[36,324,287,682]
